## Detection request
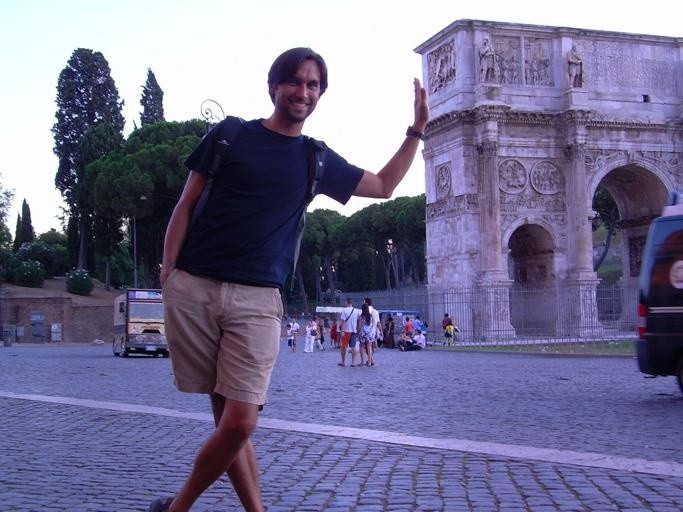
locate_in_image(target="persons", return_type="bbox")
[441,313,452,346]
[494,53,553,85]
[476,35,495,84]
[147,43,431,510]
[567,42,585,90]
[442,320,455,348]
[284,295,429,368]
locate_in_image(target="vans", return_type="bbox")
[376,310,427,325]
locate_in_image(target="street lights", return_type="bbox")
[133,194,147,288]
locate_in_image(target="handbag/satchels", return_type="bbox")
[364,329,374,341]
[445,332,452,337]
[311,330,317,335]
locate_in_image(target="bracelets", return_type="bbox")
[404,124,425,143]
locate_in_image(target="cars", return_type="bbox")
[637,215,683,395]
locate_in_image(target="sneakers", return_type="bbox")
[149,495,175,512]
[400,345,404,352]
[336,361,374,368]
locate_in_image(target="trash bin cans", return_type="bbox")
[3,324,16,347]
[50,323,63,342]
